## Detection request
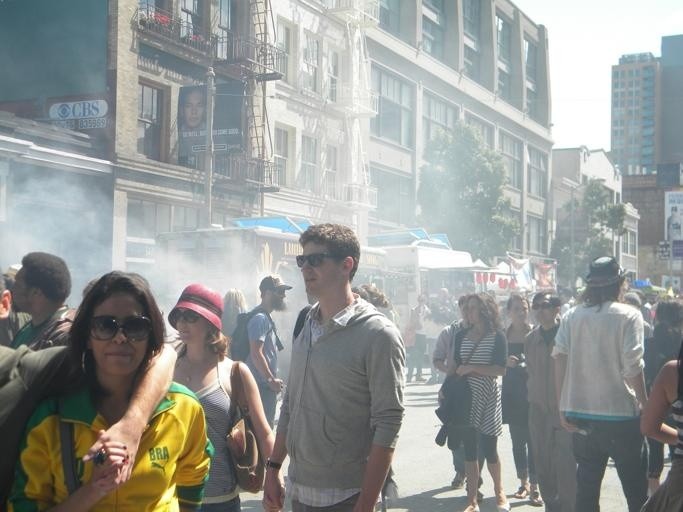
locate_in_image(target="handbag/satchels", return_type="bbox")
[435,329,501,451]
[223,361,267,494]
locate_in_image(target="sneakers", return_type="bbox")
[452,471,466,489]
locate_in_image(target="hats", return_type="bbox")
[258,275,292,297]
[167,281,225,334]
[587,255,630,286]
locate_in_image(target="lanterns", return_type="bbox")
[476,273,481,285]
[498,278,508,289]
[483,272,489,284]
[490,273,496,284]
[510,279,518,289]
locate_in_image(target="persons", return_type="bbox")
[426,288,455,385]
[350,283,400,324]
[405,293,427,383]
[642,300,682,502]
[262,223,405,511]
[233,276,293,466]
[169,285,270,512]
[454,291,508,511]
[522,290,577,512]
[219,287,246,337]
[8,251,76,351]
[7,270,214,511]
[549,257,648,512]
[640,336,683,512]
[500,292,543,508]
[177,86,207,155]
[556,284,578,318]
[0,265,177,511]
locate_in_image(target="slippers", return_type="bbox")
[514,487,530,499]
[528,491,543,507]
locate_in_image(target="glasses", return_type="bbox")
[173,310,203,324]
[530,302,555,310]
[297,252,346,270]
[85,315,152,343]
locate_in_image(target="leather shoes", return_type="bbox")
[493,491,510,512]
[469,503,480,512]
